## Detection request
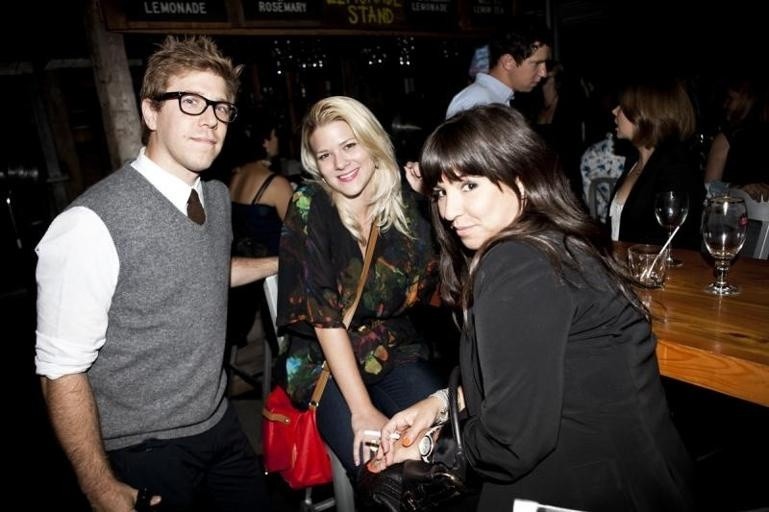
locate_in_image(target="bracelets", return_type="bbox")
[428,387,461,421]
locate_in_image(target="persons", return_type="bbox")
[366,104,704,512]
[274,88,466,481]
[28,33,308,511]
[228,0,769,260]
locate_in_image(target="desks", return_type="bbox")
[612,240,768,409]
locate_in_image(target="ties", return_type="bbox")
[187,190,205,224]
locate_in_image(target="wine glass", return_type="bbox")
[651,188,689,267]
[701,197,750,296]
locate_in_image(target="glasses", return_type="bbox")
[153,91,239,122]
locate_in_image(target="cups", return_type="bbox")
[628,244,671,289]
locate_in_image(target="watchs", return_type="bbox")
[417,421,447,464]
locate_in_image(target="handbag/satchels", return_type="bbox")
[262,385,332,489]
[354,366,464,512]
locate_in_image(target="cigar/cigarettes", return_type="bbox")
[364,427,401,440]
